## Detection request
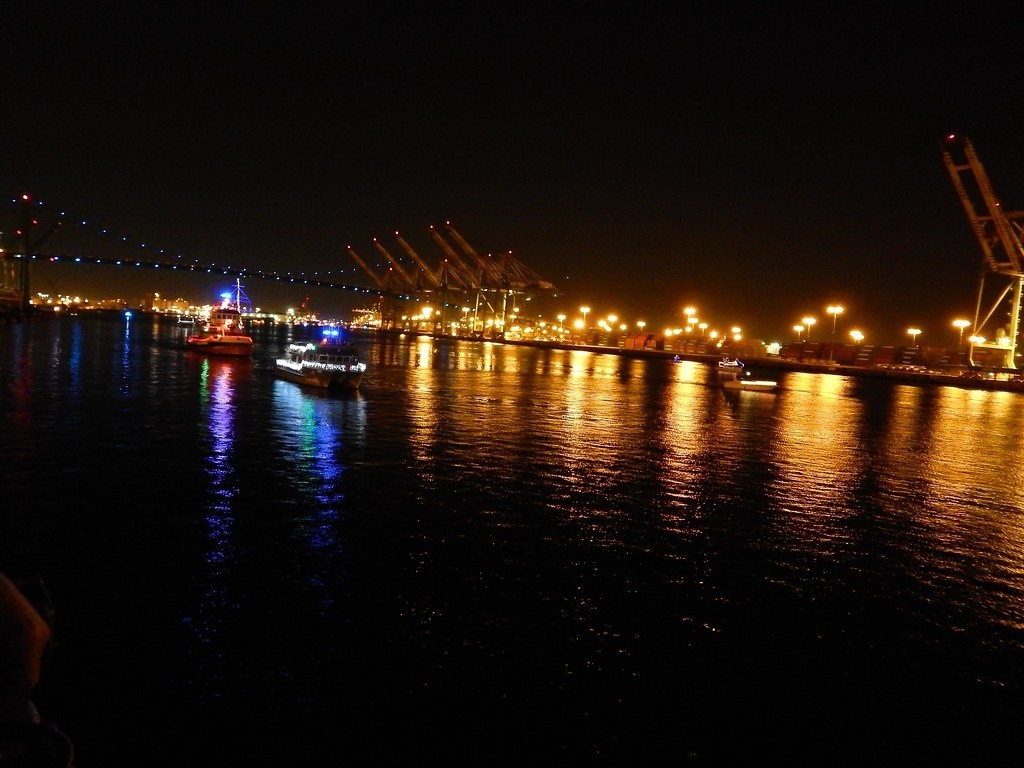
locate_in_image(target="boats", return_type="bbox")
[317,328,357,361]
[715,358,747,373]
[723,380,778,391]
[273,340,367,390]
[186,309,253,355]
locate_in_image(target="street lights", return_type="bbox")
[700,323,708,334]
[803,318,816,337]
[793,325,805,339]
[953,320,971,346]
[684,308,696,324]
[827,306,843,360]
[557,314,565,327]
[908,329,922,347]
[690,318,697,328]
[580,307,590,322]
